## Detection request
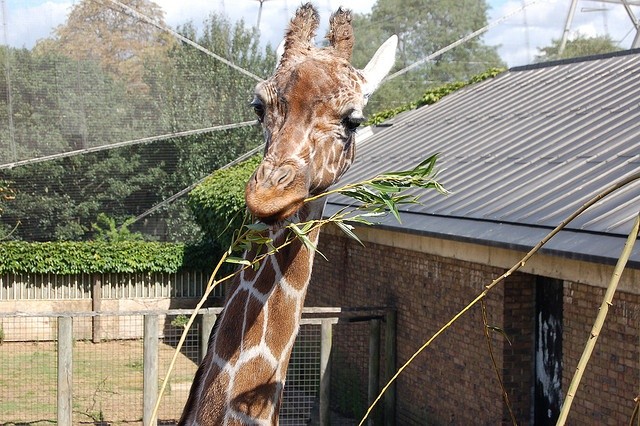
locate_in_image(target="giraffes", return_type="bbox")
[176,2,398,426]
